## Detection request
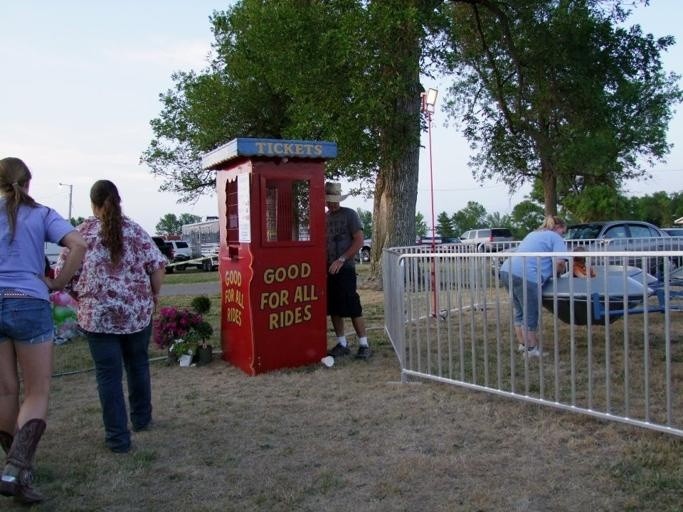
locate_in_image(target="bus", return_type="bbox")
[178,216,220,272]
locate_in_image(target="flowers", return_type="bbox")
[169,338,189,354]
[152,307,202,347]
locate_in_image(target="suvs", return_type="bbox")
[165,241,192,272]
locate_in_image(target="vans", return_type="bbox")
[151,236,174,273]
[457,227,513,252]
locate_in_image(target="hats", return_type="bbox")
[324,182,349,204]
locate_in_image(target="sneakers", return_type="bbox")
[516,344,550,360]
[354,344,372,360]
[327,340,351,358]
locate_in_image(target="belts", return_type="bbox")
[0,288,36,299]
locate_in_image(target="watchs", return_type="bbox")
[337,255,347,263]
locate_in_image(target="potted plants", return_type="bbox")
[192,296,213,363]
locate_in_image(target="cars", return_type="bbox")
[359,234,421,262]
[566,222,682,269]
[419,236,461,258]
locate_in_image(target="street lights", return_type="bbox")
[59,183,72,223]
[419,86,443,318]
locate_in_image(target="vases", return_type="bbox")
[180,356,192,367]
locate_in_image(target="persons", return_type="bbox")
[325,182,372,359]
[573,246,596,278]
[0,157,91,504]
[55,180,168,455]
[499,215,571,357]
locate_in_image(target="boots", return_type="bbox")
[0,416,48,506]
